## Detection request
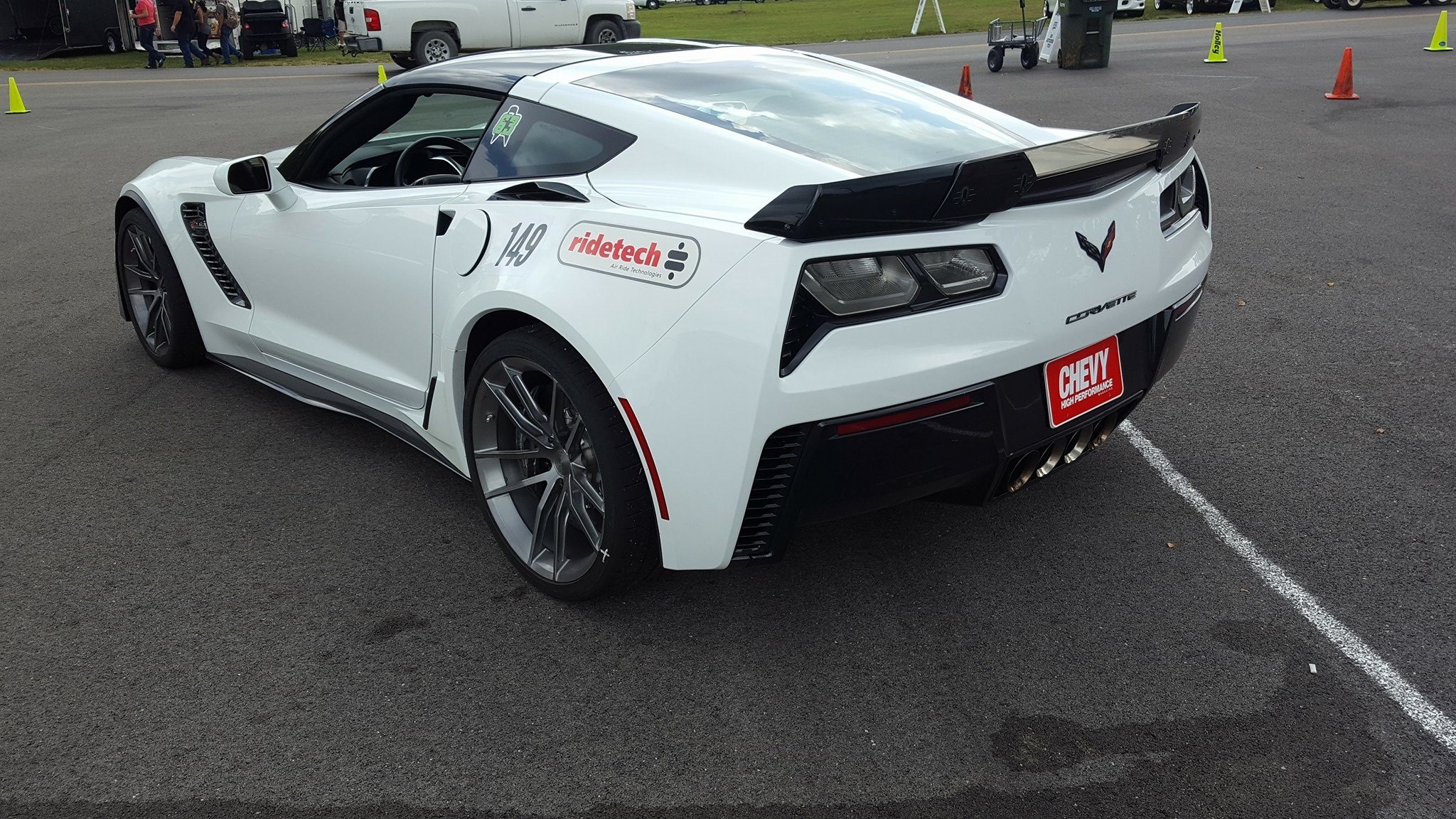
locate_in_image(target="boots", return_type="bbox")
[211,52,223,65]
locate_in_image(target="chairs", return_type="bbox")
[300,17,340,52]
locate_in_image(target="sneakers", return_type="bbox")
[146,64,159,69]
[237,53,244,62]
[222,60,232,64]
[159,55,166,66]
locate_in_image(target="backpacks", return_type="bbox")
[219,3,241,28]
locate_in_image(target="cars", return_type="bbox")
[635,0,765,11]
[1042,0,1455,21]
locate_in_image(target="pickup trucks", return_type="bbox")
[341,0,641,70]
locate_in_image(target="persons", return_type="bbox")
[334,0,348,48]
[170,0,210,68]
[129,0,167,69]
[194,0,223,66]
[215,0,244,65]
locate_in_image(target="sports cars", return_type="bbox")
[110,36,1220,605]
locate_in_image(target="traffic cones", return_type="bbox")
[5,77,31,114]
[1204,21,1227,64]
[1324,47,1361,99]
[377,65,388,84]
[959,65,973,99]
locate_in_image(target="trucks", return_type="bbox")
[32,0,127,58]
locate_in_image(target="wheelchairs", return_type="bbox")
[293,28,307,50]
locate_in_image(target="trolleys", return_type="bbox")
[987,0,1048,72]
